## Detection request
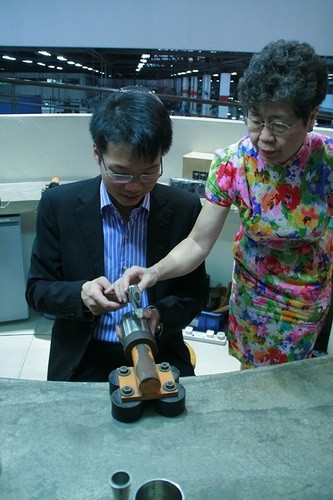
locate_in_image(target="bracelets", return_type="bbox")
[155,313,162,338]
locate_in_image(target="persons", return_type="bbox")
[104,39,332,371]
[25,90,209,382]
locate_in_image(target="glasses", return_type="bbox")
[99,150,163,184]
[244,117,303,133]
